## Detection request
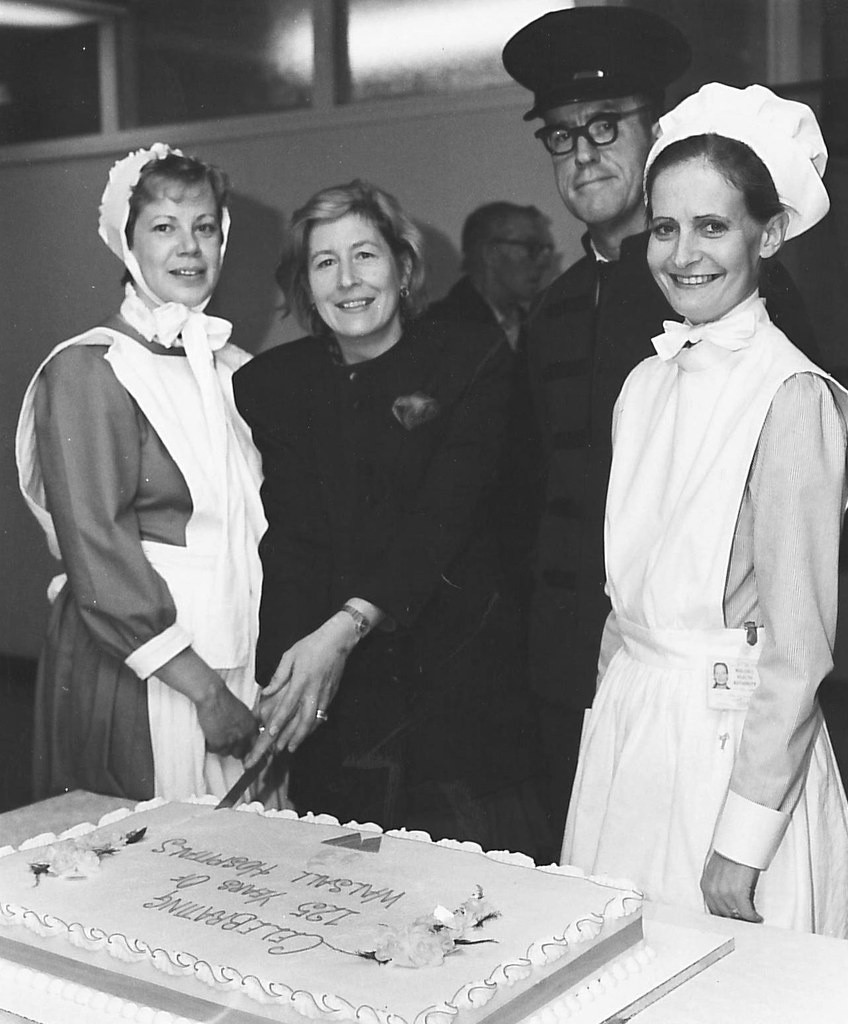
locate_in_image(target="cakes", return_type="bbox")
[0,793,646,1024]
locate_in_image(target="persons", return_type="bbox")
[15,142,295,809]
[418,7,823,866]
[232,180,507,853]
[561,80,848,937]
[713,663,731,689]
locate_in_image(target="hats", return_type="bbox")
[642,82,831,242]
[502,6,688,121]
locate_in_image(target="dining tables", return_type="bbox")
[0,789,848,1024]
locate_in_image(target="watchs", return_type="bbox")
[343,605,372,638]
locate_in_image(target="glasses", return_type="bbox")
[535,103,656,155]
[494,238,550,259]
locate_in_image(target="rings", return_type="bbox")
[260,725,266,734]
[316,709,328,721]
